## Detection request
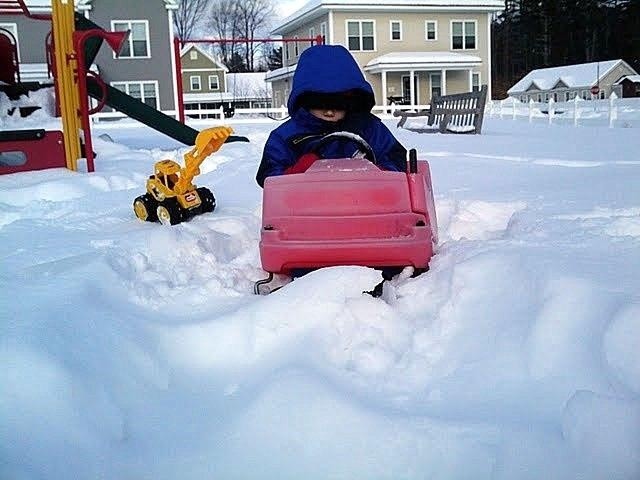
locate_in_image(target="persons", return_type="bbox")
[253,42,413,191]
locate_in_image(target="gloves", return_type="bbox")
[283,152,318,174]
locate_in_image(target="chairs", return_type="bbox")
[392,84,488,135]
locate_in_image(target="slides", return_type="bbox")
[85,75,251,146]
